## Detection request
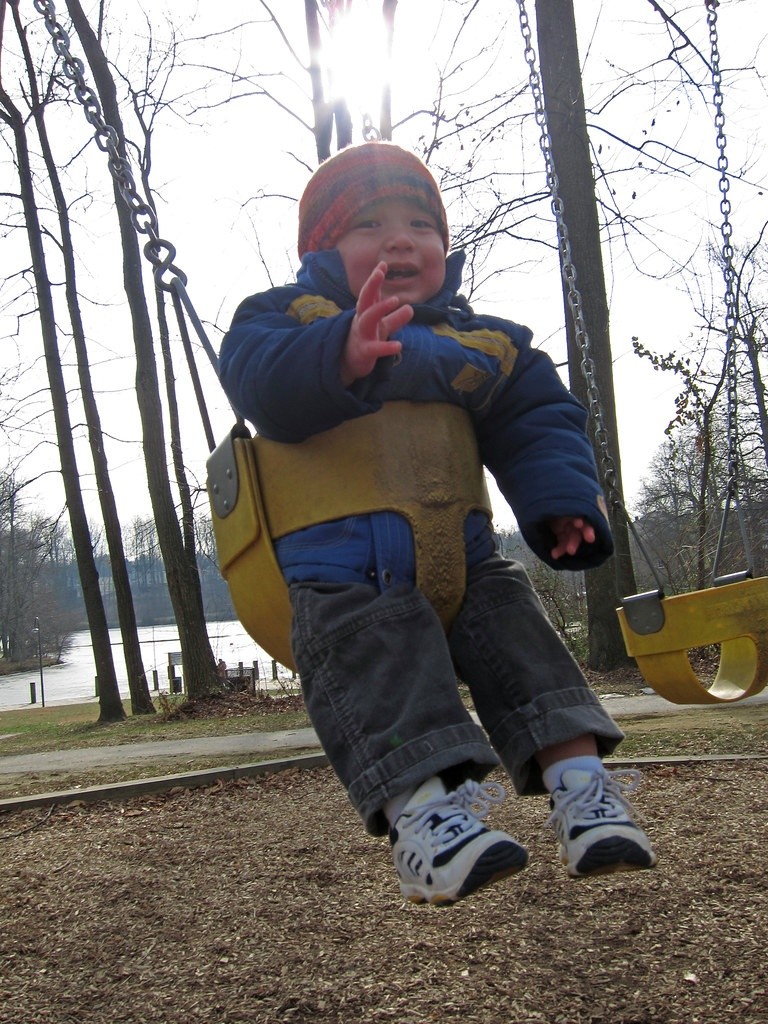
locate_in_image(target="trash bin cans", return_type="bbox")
[173,678,182,692]
[225,667,256,697]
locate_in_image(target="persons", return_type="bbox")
[220,142,658,907]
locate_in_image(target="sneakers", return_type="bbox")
[544,769,656,879]
[389,777,529,906]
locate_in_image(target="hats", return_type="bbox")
[297,143,449,263]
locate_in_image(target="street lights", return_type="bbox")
[32,616,45,708]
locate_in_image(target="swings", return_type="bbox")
[515,0,767,704]
[33,0,493,671]
